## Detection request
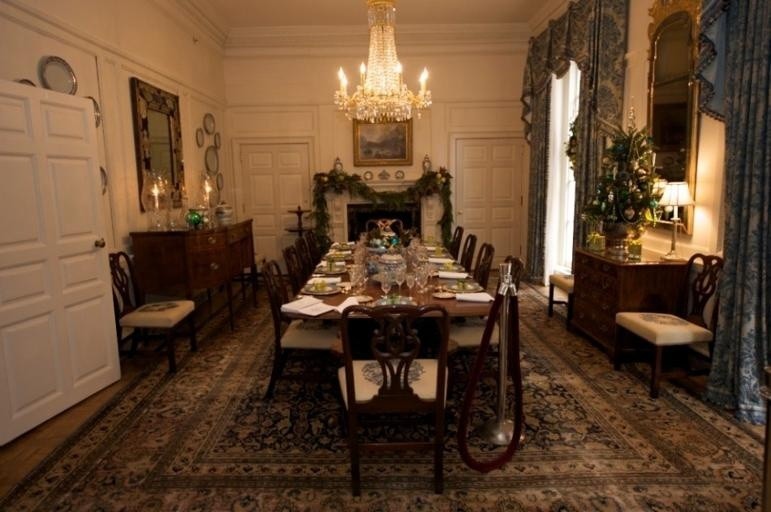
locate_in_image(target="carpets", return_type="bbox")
[0,277,770,512]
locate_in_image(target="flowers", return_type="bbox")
[579,109,665,245]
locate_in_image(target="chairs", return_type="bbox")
[614,253,724,399]
[548,275,576,332]
[108,252,198,373]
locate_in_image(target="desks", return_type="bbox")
[572,247,695,371]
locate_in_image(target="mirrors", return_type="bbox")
[642,0,702,235]
[129,77,186,213]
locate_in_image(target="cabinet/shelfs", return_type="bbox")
[129,219,258,349]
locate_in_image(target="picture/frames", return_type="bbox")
[352,118,414,166]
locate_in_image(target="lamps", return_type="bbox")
[658,181,697,262]
[198,172,220,209]
[140,169,173,231]
[333,0,433,123]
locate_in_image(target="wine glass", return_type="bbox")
[349,232,437,299]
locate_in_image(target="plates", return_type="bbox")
[19,79,37,87]
[98,167,108,196]
[196,112,224,190]
[42,55,78,96]
[395,170,405,179]
[363,171,374,180]
[300,242,483,306]
[87,96,101,129]
